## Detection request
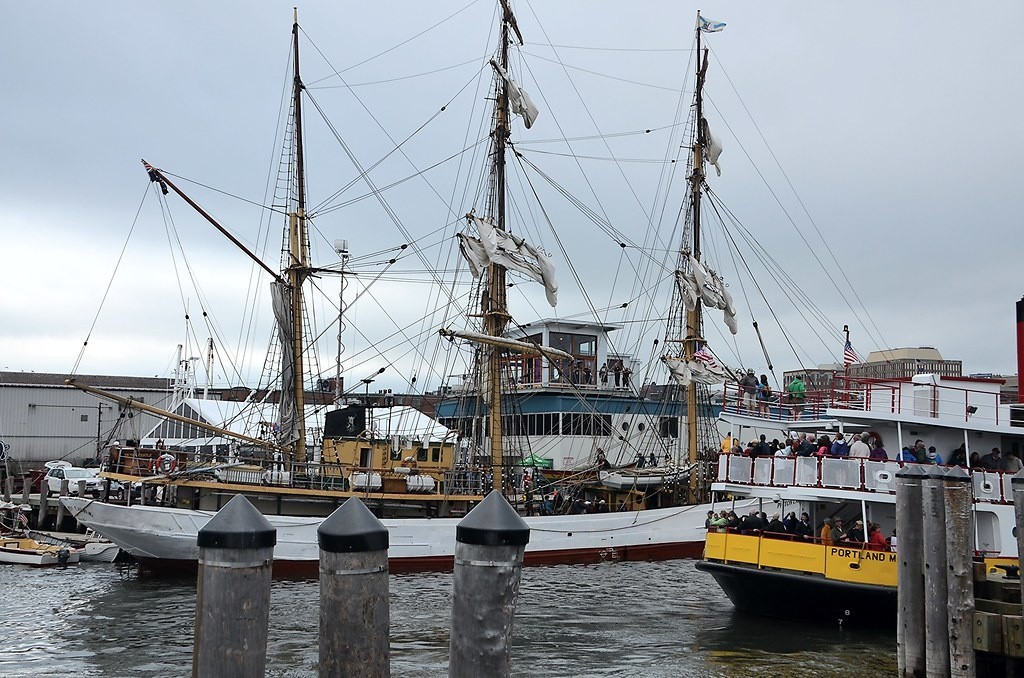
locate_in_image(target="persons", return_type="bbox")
[582,362,632,390]
[745,431,887,492]
[788,375,805,421]
[705,509,813,575]
[980,447,1023,470]
[951,443,967,466]
[722,432,743,457]
[596,448,611,471]
[648,453,657,467]
[896,440,944,465]
[757,375,770,419]
[740,369,759,417]
[637,454,645,467]
[523,472,626,514]
[821,516,896,552]
[969,452,983,468]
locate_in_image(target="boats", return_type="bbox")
[0,521,124,567]
[697,370,1024,678]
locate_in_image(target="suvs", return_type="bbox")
[43,460,101,499]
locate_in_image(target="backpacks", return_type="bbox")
[762,382,773,397]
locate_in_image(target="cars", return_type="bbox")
[87,466,119,490]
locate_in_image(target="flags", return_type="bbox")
[18,510,28,525]
[700,16,726,33]
[692,349,717,367]
[844,343,859,367]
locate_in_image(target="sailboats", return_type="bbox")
[51,0,889,582]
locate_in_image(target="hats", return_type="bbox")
[992,448,1000,454]
[916,442,925,448]
[706,511,714,515]
[748,508,759,518]
[832,516,843,524]
[721,511,726,518]
[747,368,755,375]
[790,511,795,516]
[824,518,831,524]
[856,521,863,525]
[772,512,780,517]
[1004,451,1013,456]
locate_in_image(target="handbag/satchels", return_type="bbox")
[788,390,793,400]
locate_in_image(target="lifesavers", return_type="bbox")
[401,456,417,468]
[149,457,152,473]
[156,454,176,474]
